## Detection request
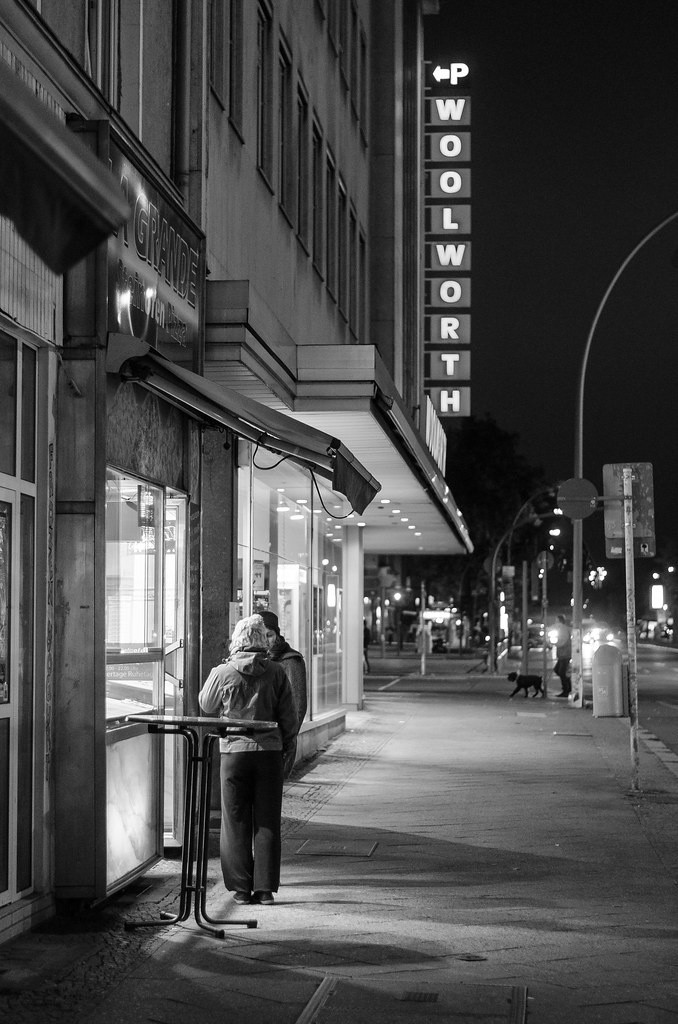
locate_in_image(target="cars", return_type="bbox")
[581,619,627,644]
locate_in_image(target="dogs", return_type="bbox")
[506,672,546,698]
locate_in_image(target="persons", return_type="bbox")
[363,620,372,675]
[197,613,298,905]
[552,614,572,697]
[258,611,307,753]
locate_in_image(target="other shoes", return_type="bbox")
[557,687,571,698]
[233,891,251,905]
[253,890,274,905]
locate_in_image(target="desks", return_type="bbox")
[124,715,278,938]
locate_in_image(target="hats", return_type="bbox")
[232,613,266,647]
[258,612,280,635]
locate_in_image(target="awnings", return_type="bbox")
[139,352,383,516]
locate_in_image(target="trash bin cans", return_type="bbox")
[592,644,625,717]
